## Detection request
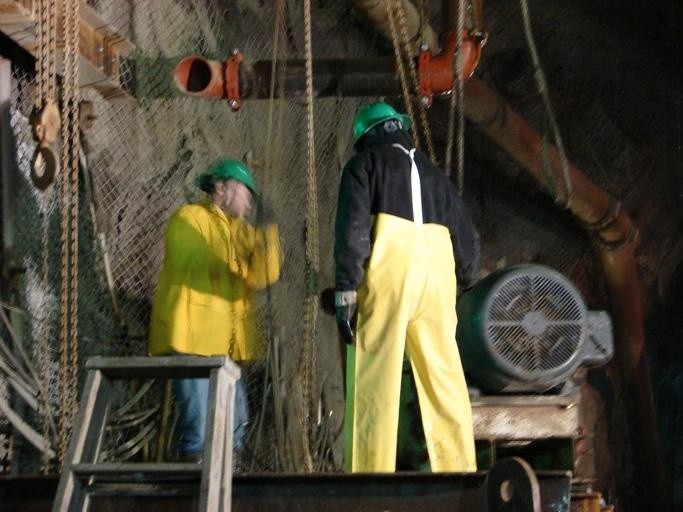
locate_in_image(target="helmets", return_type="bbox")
[352,101,412,136]
[194,159,258,196]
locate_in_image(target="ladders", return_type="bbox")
[53,354,240,512]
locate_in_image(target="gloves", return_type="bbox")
[336,290,359,344]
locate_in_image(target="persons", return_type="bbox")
[144,157,284,478]
[333,101,482,475]
